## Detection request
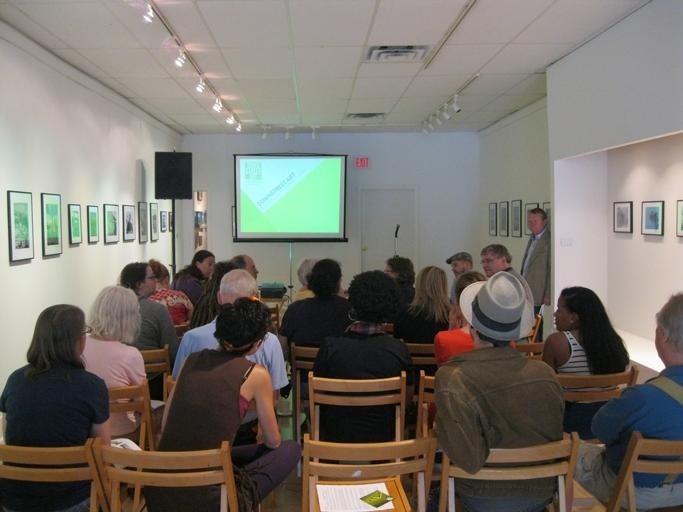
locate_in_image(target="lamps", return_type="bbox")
[452,94,462,114]
[420,126,430,138]
[427,118,436,132]
[310,126,320,139]
[439,103,452,121]
[283,127,293,140]
[435,109,444,127]
[261,124,270,142]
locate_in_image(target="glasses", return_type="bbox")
[147,274,157,280]
[219,337,255,352]
[80,325,93,335]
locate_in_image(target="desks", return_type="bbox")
[263,298,286,305]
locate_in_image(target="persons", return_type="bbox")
[573,293,683,512]
[172,269,289,391]
[121,262,179,401]
[542,287,630,440]
[80,286,146,445]
[190,262,234,329]
[394,266,451,343]
[384,257,416,303]
[231,255,261,280]
[434,272,486,368]
[311,270,415,444]
[0,304,111,511]
[480,244,533,310]
[279,258,353,399]
[433,271,566,512]
[447,251,473,303]
[145,297,302,512]
[170,251,215,304]
[279,258,321,325]
[145,260,193,325]
[520,208,550,343]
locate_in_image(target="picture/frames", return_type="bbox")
[641,200,665,236]
[498,201,509,237]
[488,203,498,237]
[103,203,120,244]
[40,193,63,257]
[160,211,168,232]
[138,202,149,243]
[524,203,539,235]
[169,212,175,233]
[68,204,83,244]
[612,201,633,233]
[676,200,682,238]
[6,190,34,262]
[510,200,522,238]
[192,192,208,251]
[149,203,160,242]
[122,204,138,241]
[87,205,100,243]
[543,202,552,232]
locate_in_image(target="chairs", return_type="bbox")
[140,345,174,402]
[402,340,435,405]
[302,433,437,512]
[308,371,406,479]
[176,323,190,343]
[270,303,285,331]
[92,437,238,512]
[439,431,578,511]
[607,431,683,511]
[514,344,545,361]
[291,340,320,393]
[0,438,99,512]
[416,367,443,437]
[556,365,639,407]
[528,314,541,343]
[107,382,154,451]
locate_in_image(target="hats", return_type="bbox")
[459,271,534,342]
[446,251,472,264]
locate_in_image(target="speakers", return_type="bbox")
[155,152,193,201]
